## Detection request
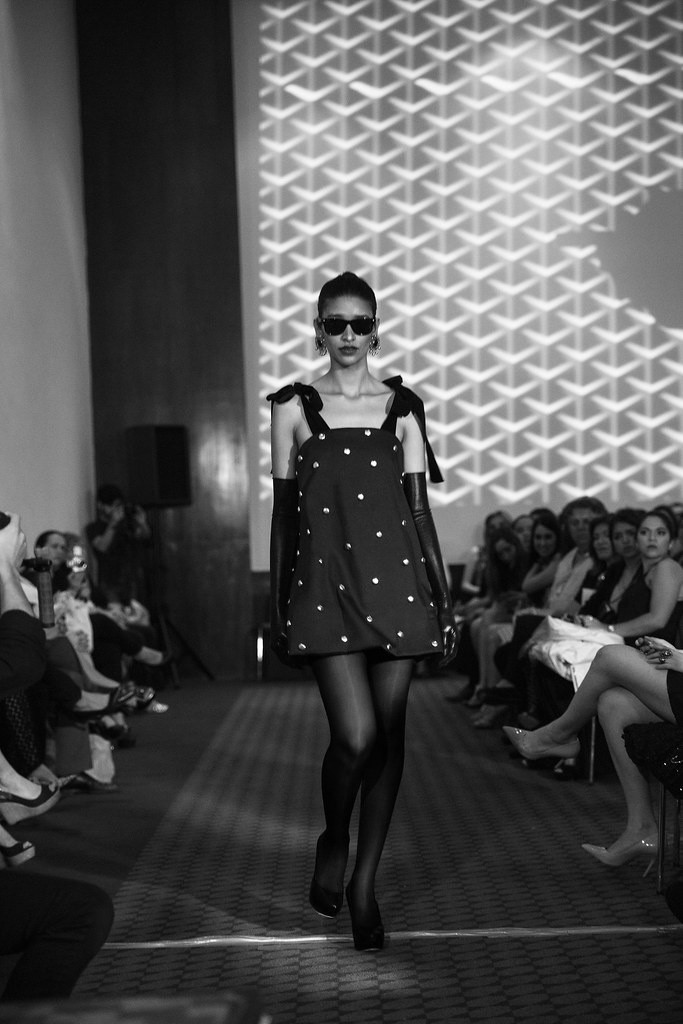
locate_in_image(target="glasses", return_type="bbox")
[317,317,375,336]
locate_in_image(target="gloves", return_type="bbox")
[403,472,460,668]
[270,477,312,670]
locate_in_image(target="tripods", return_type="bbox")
[147,507,217,692]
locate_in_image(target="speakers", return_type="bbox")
[125,425,193,508]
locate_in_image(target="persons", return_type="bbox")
[446,495,683,879]
[266,271,461,950]
[84,482,170,714]
[1,512,170,873]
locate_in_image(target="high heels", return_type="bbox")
[582,833,659,878]
[503,726,581,768]
[0,841,36,870]
[0,781,61,825]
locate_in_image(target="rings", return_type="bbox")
[659,657,665,663]
[660,650,671,658]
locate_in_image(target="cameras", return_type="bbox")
[67,546,87,572]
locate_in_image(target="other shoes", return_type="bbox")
[60,648,177,792]
[346,886,384,952]
[444,681,538,749]
[551,750,598,778]
[309,830,351,919]
[520,758,553,769]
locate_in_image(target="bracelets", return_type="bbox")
[608,622,615,633]
